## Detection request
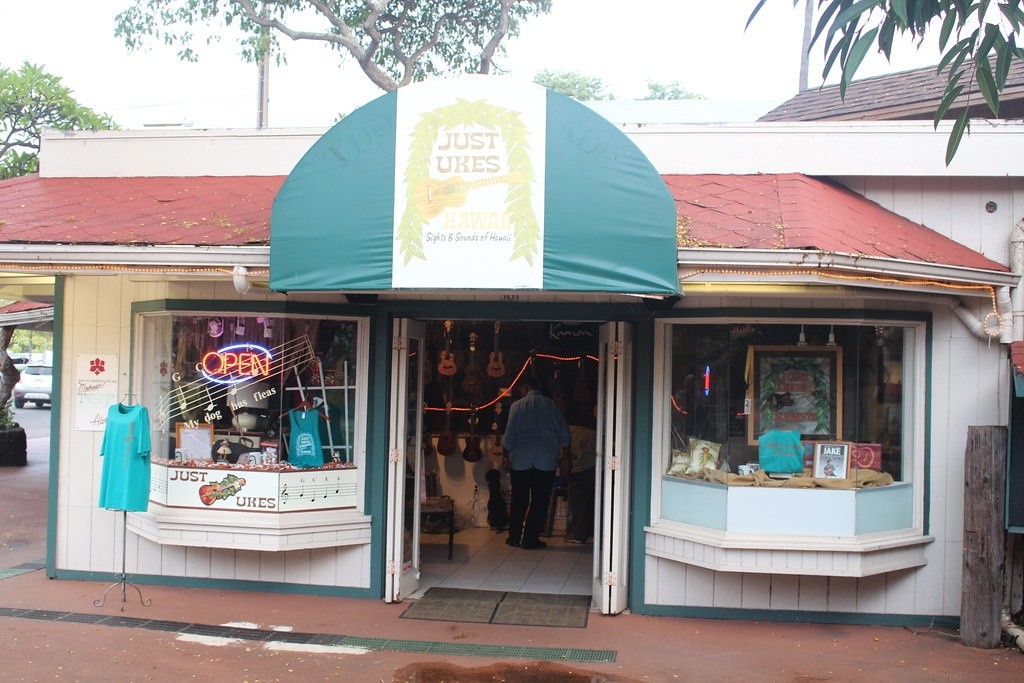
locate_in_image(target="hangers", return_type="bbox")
[120,391,139,405]
[278,390,332,423]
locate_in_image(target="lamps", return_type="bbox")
[235,317,275,337]
[826,325,837,347]
[797,325,808,347]
[208,316,224,337]
[226,382,270,431]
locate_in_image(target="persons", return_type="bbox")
[500,371,573,549]
[559,423,596,543]
[824,459,837,477]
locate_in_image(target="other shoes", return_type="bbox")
[564,534,583,544]
[506,536,521,546]
[520,540,547,550]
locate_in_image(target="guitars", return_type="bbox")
[437,319,458,376]
[462,403,482,462]
[486,321,506,377]
[461,333,482,392]
[767,389,821,412]
[437,401,458,456]
[824,464,840,476]
[485,403,507,465]
[525,348,592,405]
[414,173,521,218]
[421,402,433,455]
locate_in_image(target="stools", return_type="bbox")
[421,494,454,559]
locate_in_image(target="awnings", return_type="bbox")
[270,73,685,303]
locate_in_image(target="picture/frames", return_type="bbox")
[811,440,852,479]
[176,421,214,461]
[747,345,843,446]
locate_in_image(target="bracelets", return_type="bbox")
[502,458,509,461]
